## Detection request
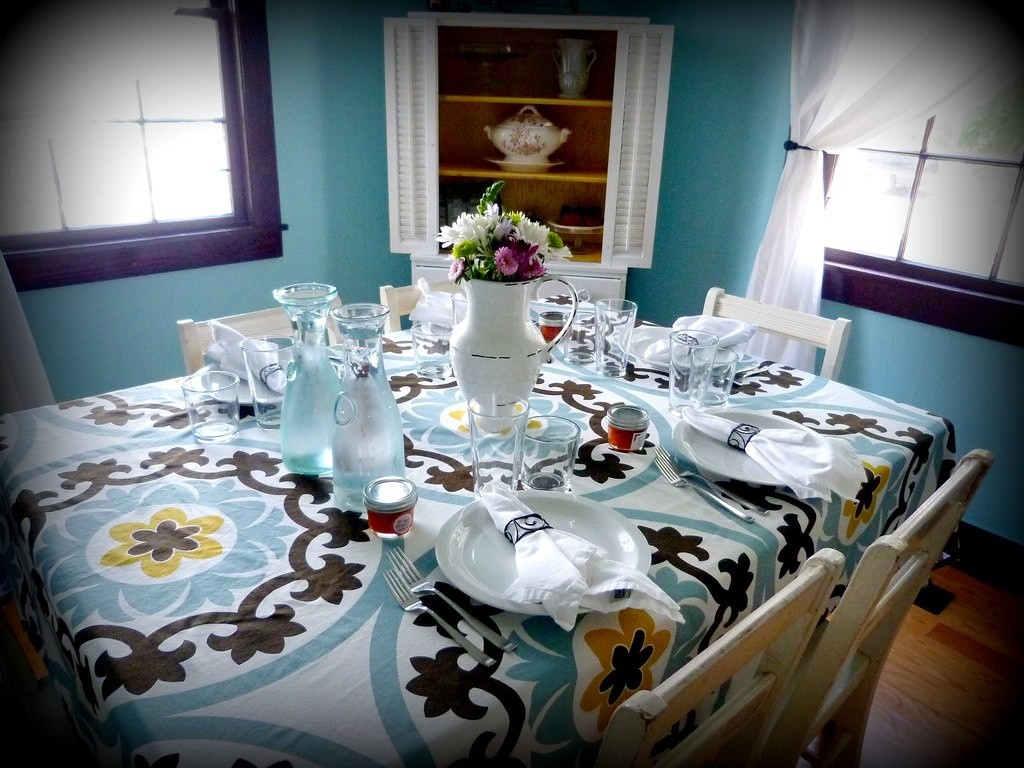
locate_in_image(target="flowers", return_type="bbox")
[436,181,572,289]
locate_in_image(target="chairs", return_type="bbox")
[379,279,462,332]
[698,287,853,380]
[591,548,845,768]
[765,445,1001,767]
[177,295,342,373]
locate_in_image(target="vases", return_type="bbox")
[445,275,580,412]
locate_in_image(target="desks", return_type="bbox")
[0,319,957,767]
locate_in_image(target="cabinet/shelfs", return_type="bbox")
[383,8,675,268]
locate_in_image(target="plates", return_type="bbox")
[193,361,283,406]
[618,327,744,368]
[435,491,651,616]
[483,156,567,172]
[673,412,831,486]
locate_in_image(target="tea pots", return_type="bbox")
[483,105,572,164]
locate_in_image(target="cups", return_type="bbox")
[411,322,457,378]
[240,333,295,431]
[181,370,242,444]
[562,309,609,365]
[593,298,640,377]
[703,347,741,413]
[515,414,581,494]
[450,291,468,326]
[467,392,531,495]
[667,329,721,418]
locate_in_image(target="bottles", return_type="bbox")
[273,282,339,474]
[331,303,404,511]
[552,38,597,98]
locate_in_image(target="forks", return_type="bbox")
[381,567,497,668]
[385,545,519,653]
[650,445,770,524]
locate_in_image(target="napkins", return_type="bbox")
[480,479,685,628]
[643,312,760,363]
[206,317,287,396]
[671,405,869,502]
